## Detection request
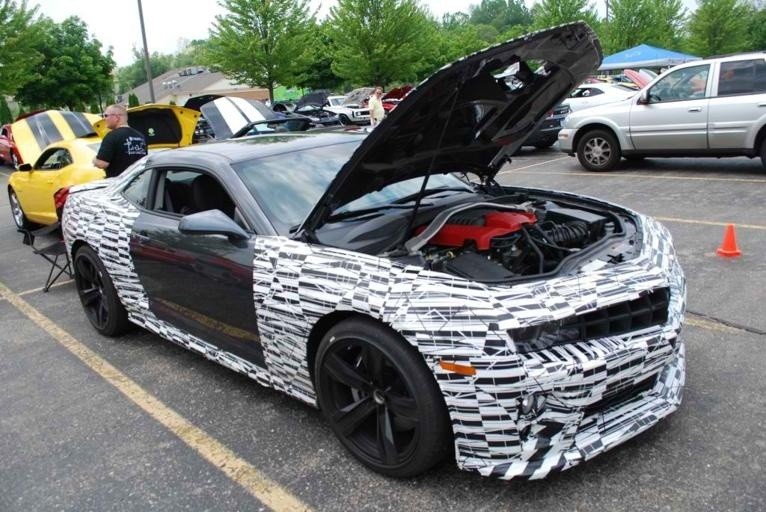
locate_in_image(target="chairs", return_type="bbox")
[191,173,235,219]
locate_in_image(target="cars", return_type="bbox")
[557,50,765,173]
[272,83,413,124]
[512,80,636,151]
[54,21,687,481]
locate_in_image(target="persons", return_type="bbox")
[92,104,148,179]
[368,87,385,127]
